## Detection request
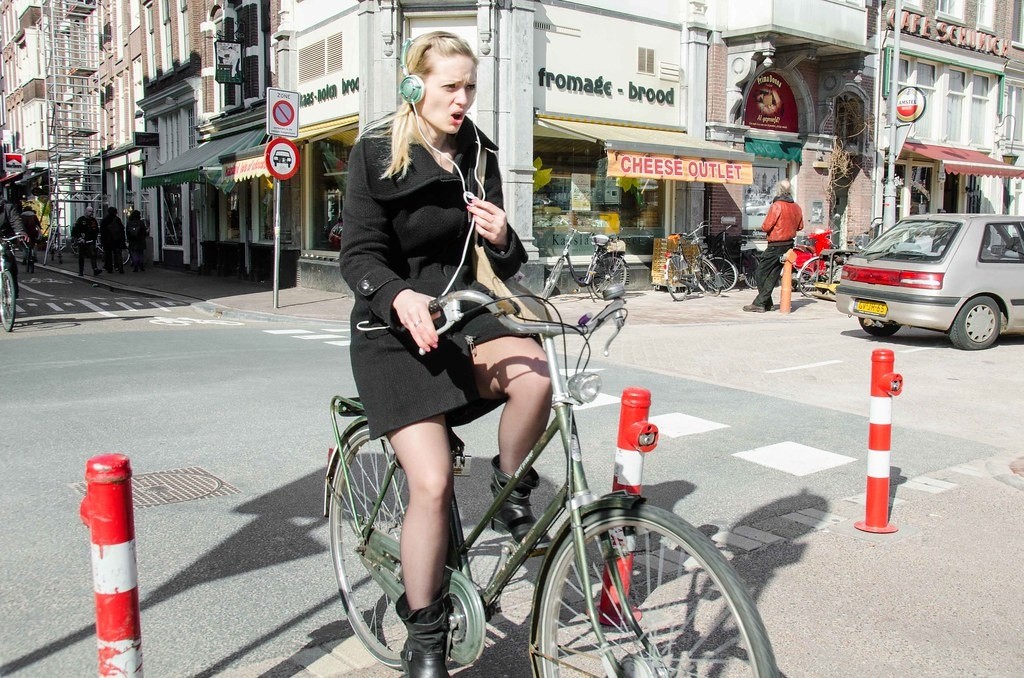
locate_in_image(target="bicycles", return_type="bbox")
[665,220,722,301]
[541,216,627,300]
[70,236,131,266]
[796,225,879,298]
[325,288,778,678]
[0,232,30,332]
[22,239,36,273]
[701,222,763,292]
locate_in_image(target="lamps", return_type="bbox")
[762,56,773,67]
[854,74,863,84]
[135,110,146,119]
[165,96,179,105]
[994,114,1019,166]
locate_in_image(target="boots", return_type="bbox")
[395,589,454,678]
[489,454,551,549]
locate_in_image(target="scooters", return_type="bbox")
[779,212,841,292]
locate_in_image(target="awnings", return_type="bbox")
[218,115,358,185]
[534,118,754,187]
[0,170,25,185]
[140,118,269,190]
[902,142,1024,181]
[15,169,50,186]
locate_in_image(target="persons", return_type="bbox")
[741,178,804,313]
[0,185,30,299]
[100,207,127,275]
[166,215,182,245]
[125,210,147,272]
[340,32,554,678]
[19,206,41,263]
[70,206,100,280]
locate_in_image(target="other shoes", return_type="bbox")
[132,268,138,272]
[21,260,27,265]
[140,268,145,271]
[743,304,765,313]
[765,305,775,311]
[33,257,38,263]
[120,270,125,274]
[114,268,118,271]
[79,272,84,276]
[93,269,102,276]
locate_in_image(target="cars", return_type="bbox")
[836,213,1024,351]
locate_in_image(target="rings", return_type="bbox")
[414,319,422,327]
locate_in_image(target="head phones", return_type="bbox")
[401,38,425,103]
